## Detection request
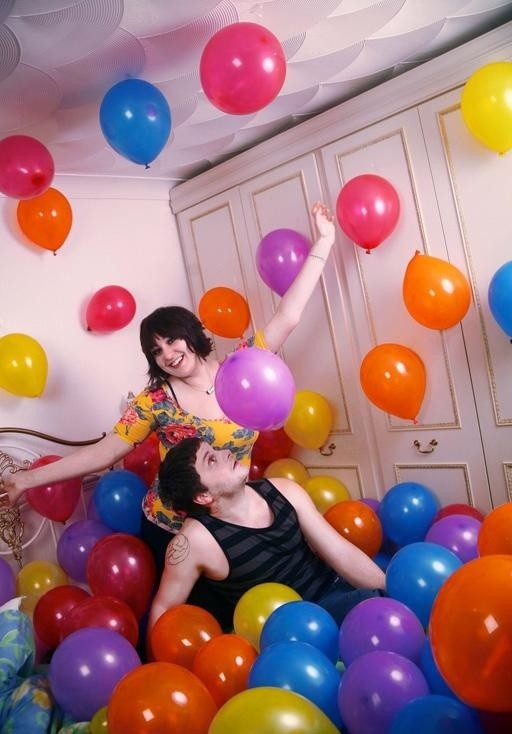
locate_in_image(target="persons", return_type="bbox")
[147,437,385,632]
[0,201,337,562]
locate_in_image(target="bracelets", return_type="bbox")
[308,254,328,263]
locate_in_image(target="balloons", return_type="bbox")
[425,515,482,563]
[429,556,512,712]
[198,287,250,340]
[192,634,258,711]
[151,605,222,670]
[248,641,340,725]
[300,475,350,515]
[403,250,472,331]
[0,558,16,606]
[16,187,73,256]
[324,500,382,556]
[16,560,69,615]
[263,458,310,484]
[432,504,483,522]
[108,662,218,734]
[57,520,113,583]
[27,455,81,525]
[488,261,512,343]
[340,597,426,664]
[255,228,313,298]
[461,62,512,154]
[87,533,155,622]
[336,174,400,254]
[208,687,340,734]
[233,582,302,652]
[250,464,262,480]
[388,696,485,734]
[33,585,90,648]
[252,427,293,462]
[0,134,55,200]
[89,706,108,734]
[477,501,512,556]
[124,437,161,486]
[0,333,48,399]
[86,285,136,333]
[49,626,142,721]
[99,79,171,170]
[92,469,148,535]
[360,343,427,424]
[338,651,428,734]
[385,542,463,629]
[377,482,439,547]
[359,498,380,512]
[284,390,332,452]
[60,594,138,647]
[214,348,296,431]
[260,601,340,663]
[200,22,286,116]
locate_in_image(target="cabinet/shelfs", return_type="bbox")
[170,23,512,520]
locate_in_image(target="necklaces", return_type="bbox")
[185,358,214,394]
